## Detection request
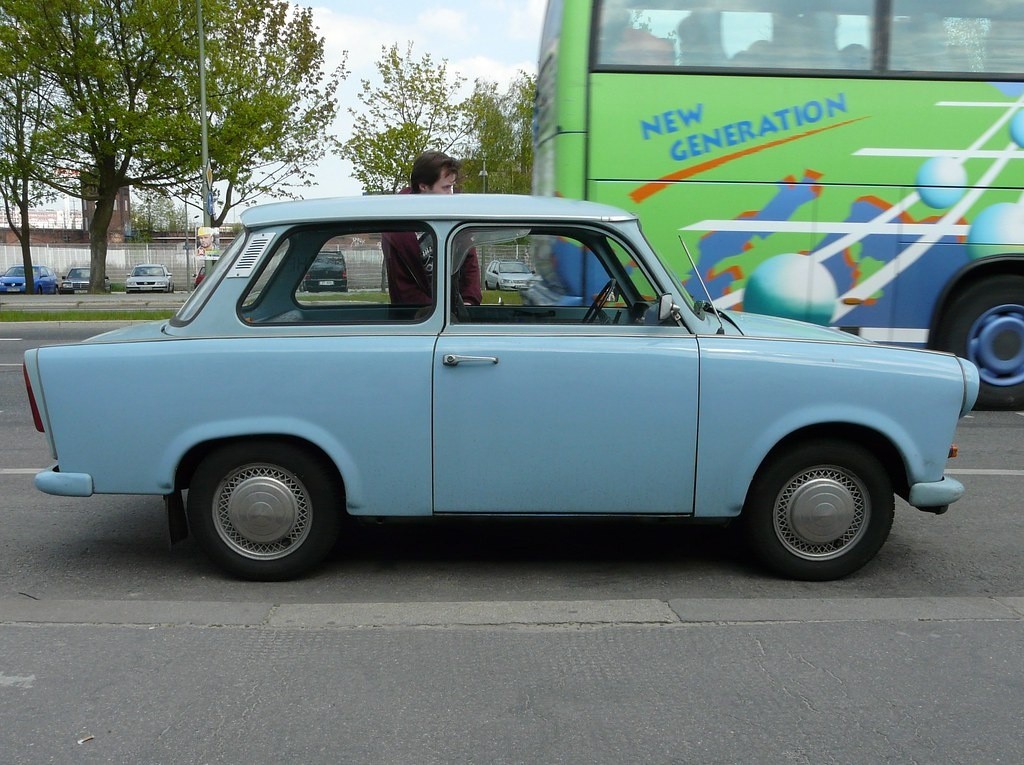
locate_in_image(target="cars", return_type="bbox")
[125,265,174,294]
[0,264,59,295]
[191,268,206,290]
[23,191,984,586]
[485,257,536,291]
[58,267,111,295]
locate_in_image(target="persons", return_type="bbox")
[197,227,218,256]
[382,149,481,308]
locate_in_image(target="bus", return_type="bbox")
[534,0,1023,418]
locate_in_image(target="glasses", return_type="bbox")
[431,183,454,193]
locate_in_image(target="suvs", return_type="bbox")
[298,251,349,293]
